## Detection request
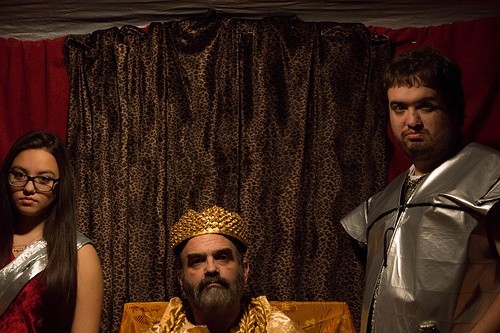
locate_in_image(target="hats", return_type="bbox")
[170,205,247,258]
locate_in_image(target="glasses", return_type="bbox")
[6,171,61,192]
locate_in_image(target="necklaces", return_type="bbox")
[12,231,43,252]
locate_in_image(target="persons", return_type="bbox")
[339,42,499,333]
[144,203,300,333]
[0,130,104,333]
